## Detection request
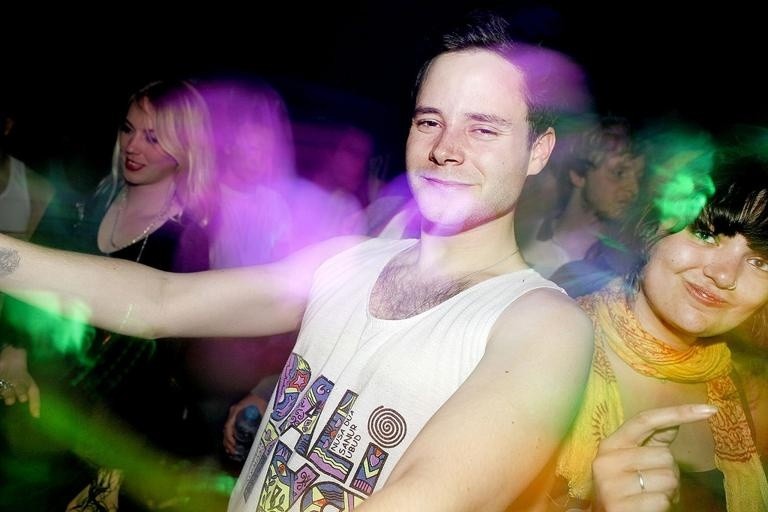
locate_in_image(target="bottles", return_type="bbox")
[228,405,263,462]
